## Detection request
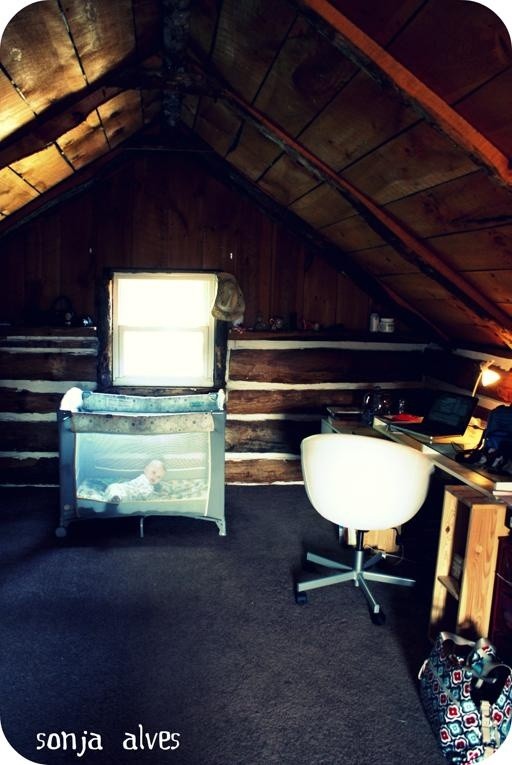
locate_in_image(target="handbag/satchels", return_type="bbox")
[418,630,512,763]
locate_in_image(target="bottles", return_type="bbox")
[369,313,380,332]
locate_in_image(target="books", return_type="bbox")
[326,407,364,429]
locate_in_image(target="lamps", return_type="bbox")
[471,359,500,398]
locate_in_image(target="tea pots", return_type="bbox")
[360,384,391,422]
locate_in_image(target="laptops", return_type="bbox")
[388,388,479,440]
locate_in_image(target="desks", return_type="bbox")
[319,405,512,637]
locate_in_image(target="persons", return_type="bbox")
[105,459,167,502]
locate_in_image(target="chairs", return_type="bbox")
[294,433,435,624]
